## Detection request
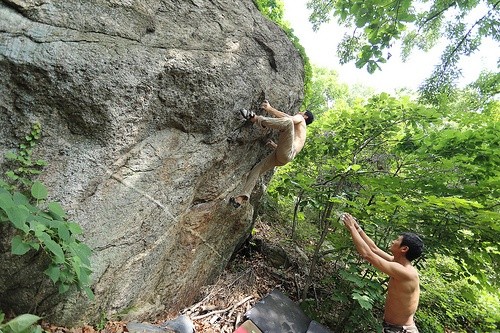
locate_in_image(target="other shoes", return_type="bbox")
[229,196,241,208]
[240,107,256,124]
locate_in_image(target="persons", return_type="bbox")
[230,99,315,209]
[342,211,428,333]
